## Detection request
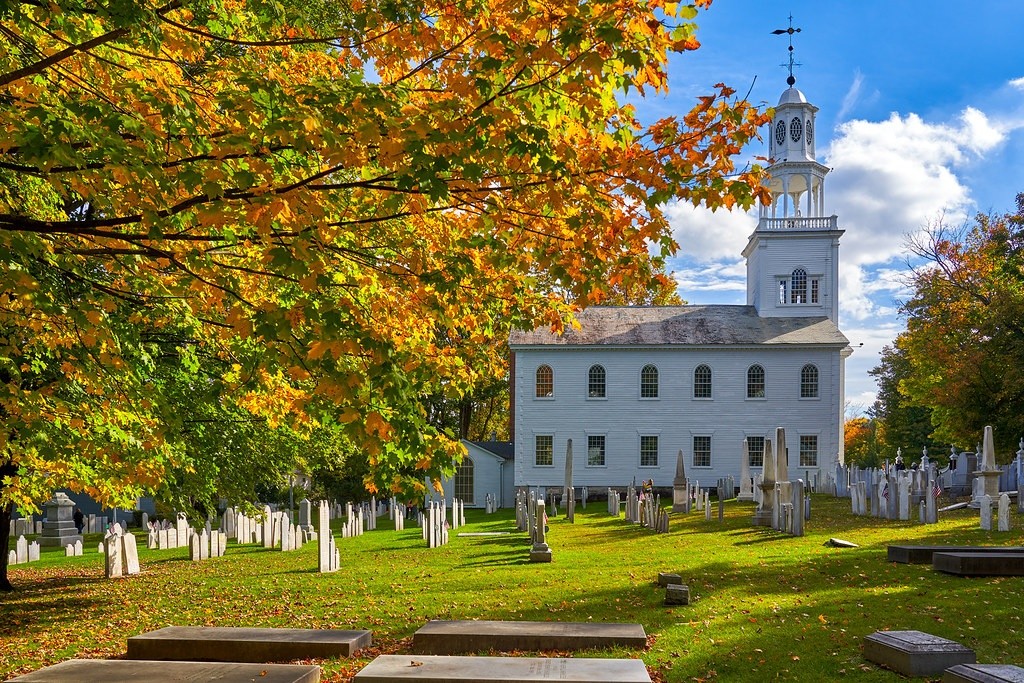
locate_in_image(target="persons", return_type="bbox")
[642,478,653,508]
[896,459,905,471]
[911,462,920,470]
[74,509,83,533]
